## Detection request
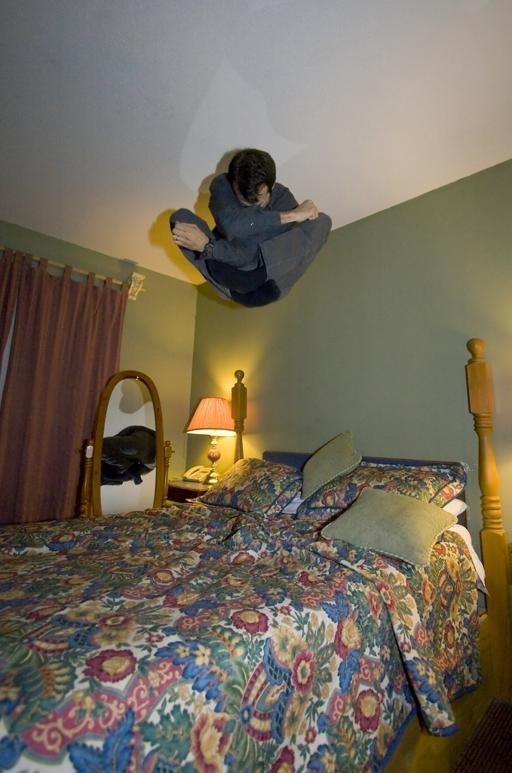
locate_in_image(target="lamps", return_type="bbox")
[185,397,236,485]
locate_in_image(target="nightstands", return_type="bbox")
[168,480,211,504]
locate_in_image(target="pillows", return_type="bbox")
[321,487,458,568]
[297,462,467,521]
[443,499,467,516]
[301,431,362,502]
[201,459,304,520]
[282,491,305,514]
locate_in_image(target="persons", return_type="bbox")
[168,148,333,306]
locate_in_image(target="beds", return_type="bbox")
[0,339,511,771]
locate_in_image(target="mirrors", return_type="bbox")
[84,370,171,519]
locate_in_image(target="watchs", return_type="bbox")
[200,237,215,257]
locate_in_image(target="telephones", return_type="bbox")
[181,465,214,483]
